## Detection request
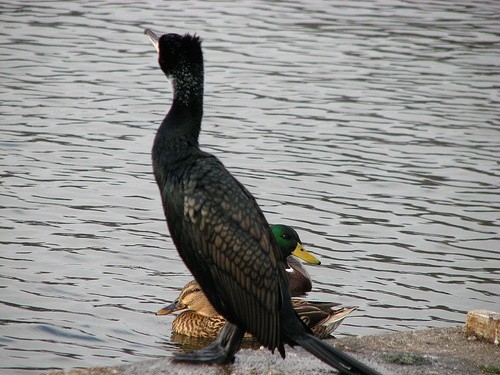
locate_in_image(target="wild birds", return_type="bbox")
[142,28,381,375]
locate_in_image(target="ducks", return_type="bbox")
[154,281,367,343]
[267,224,322,291]
